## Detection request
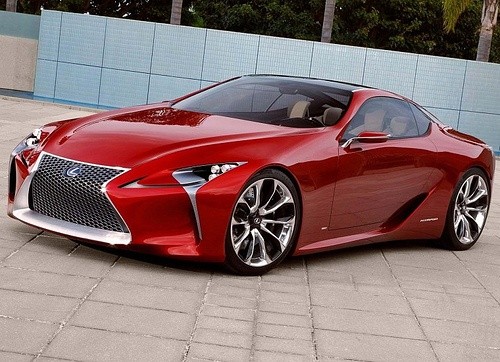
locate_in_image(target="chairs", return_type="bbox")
[322,106,343,126]
[286,100,312,119]
[386,115,411,135]
[364,108,388,132]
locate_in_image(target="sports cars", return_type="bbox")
[6,73,496,275]
